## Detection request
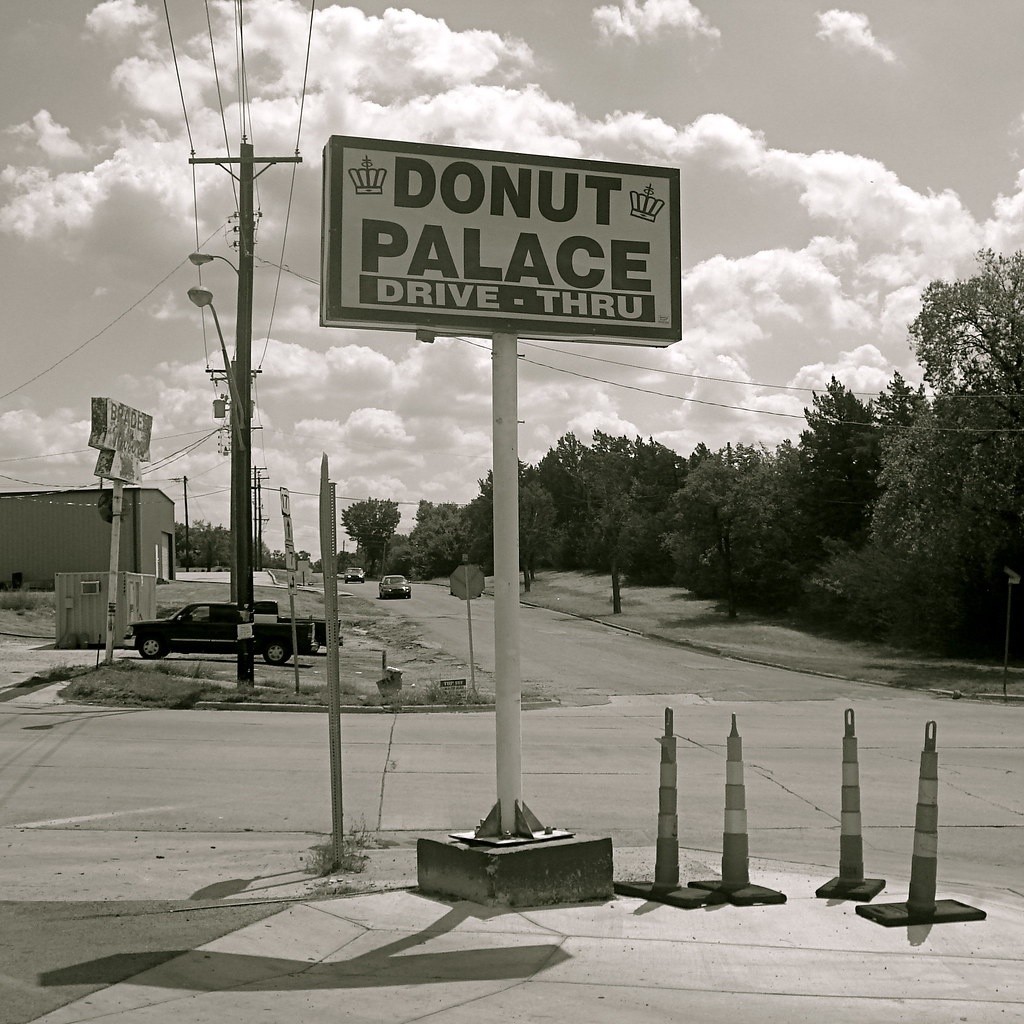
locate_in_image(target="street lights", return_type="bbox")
[187,251,254,693]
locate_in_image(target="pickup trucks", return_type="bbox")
[122,601,344,666]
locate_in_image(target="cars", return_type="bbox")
[378,574,412,600]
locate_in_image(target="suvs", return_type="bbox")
[344,567,366,583]
